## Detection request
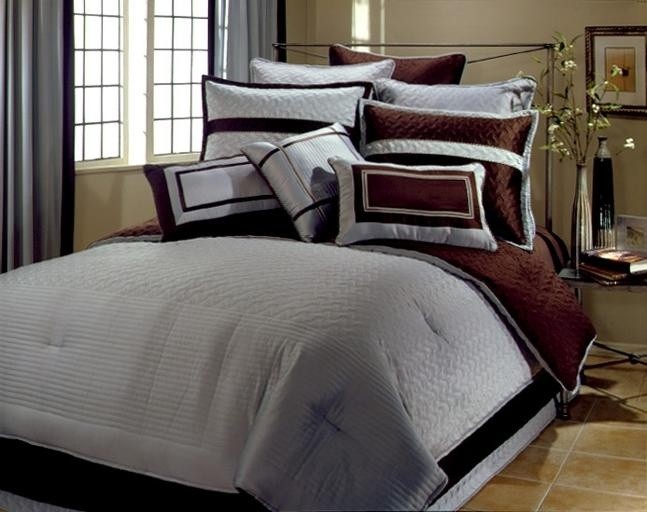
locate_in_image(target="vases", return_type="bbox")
[590,135,616,250]
[570,161,593,276]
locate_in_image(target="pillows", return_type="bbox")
[359,98,538,253]
[249,58,394,82]
[330,42,465,86]
[143,154,286,242]
[327,156,498,252]
[199,73,376,164]
[374,74,537,111]
[235,121,364,244]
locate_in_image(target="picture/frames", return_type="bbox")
[616,214,647,255]
[585,25,647,118]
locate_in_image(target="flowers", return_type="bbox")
[516,29,636,167]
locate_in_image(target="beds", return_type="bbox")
[0,43,598,512]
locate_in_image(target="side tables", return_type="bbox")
[559,265,647,418]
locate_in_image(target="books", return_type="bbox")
[579,246,646,275]
[577,264,622,280]
[580,261,627,277]
